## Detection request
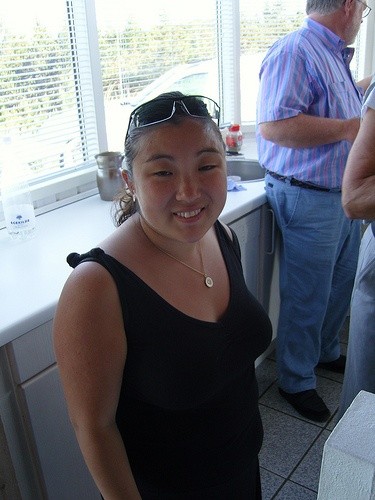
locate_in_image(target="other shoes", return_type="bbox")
[278,383,330,421]
[317,354,346,373]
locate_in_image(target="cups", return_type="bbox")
[0,176,36,240]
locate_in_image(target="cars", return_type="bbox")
[66,59,212,167]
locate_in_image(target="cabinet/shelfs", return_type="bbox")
[0,193,281,500]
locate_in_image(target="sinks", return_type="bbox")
[226,159,266,183]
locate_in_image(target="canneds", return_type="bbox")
[225,124,243,152]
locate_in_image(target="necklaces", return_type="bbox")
[151,237,213,287]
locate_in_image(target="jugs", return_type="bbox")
[94,151,125,201]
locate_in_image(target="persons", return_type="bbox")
[257,0,375,422]
[52,91,274,500]
[342,70,375,422]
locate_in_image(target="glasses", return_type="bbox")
[356,0,371,18]
[125,95,220,139]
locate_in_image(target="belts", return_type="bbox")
[266,167,342,194]
